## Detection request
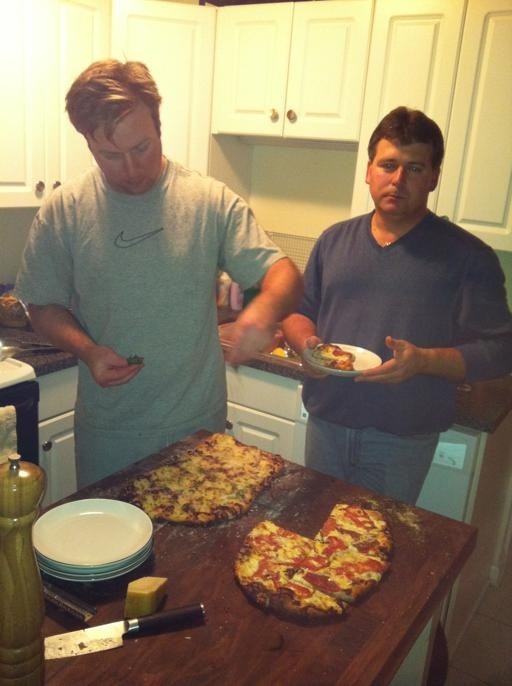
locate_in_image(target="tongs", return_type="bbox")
[0,337,64,359]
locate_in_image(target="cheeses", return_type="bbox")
[124,576,168,617]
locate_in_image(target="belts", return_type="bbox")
[316,410,454,437]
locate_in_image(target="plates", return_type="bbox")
[31,498,155,583]
[304,343,382,379]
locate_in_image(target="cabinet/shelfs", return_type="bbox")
[38,359,490,526]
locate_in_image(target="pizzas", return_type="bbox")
[232,503,392,618]
[132,432,284,526]
[311,343,355,370]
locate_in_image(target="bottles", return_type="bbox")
[217,272,258,310]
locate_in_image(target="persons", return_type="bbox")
[278,103,512,511]
[12,58,306,494]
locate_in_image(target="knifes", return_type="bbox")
[43,603,204,660]
